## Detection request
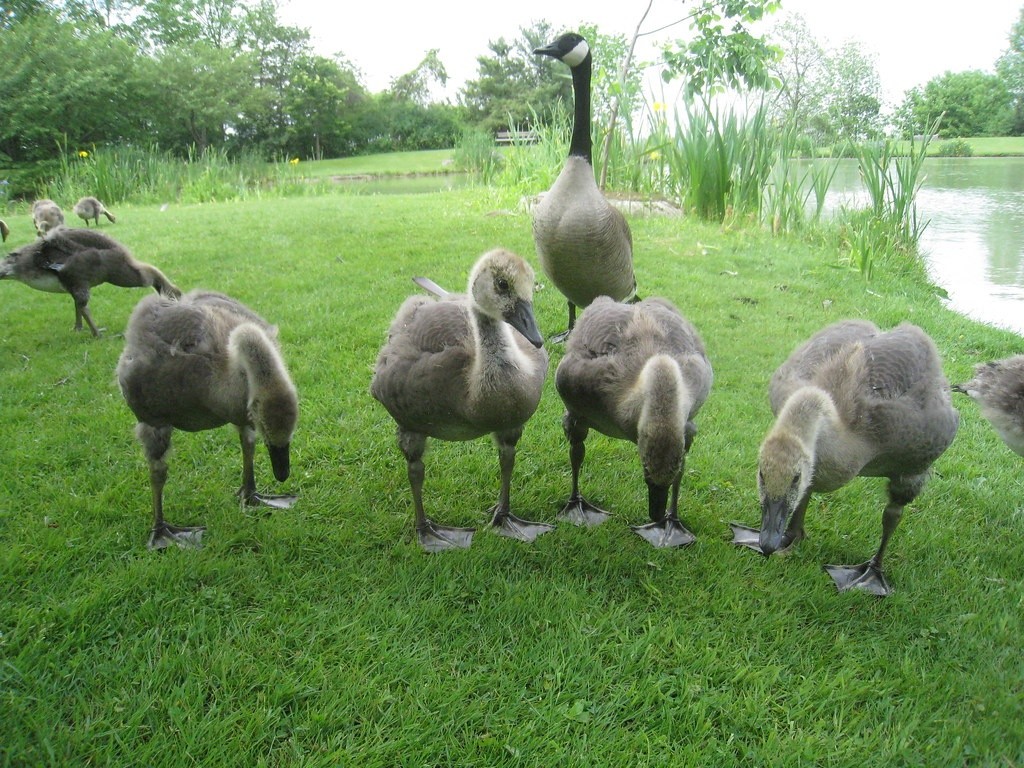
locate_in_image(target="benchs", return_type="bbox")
[494,130,535,146]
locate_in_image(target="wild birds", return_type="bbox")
[33,199,63,237]
[0,220,9,243]
[727,317,962,595]
[555,293,698,549]
[0,225,183,341]
[115,290,298,550]
[533,32,642,347]
[952,350,1024,458]
[369,247,558,553]
[72,197,116,227]
